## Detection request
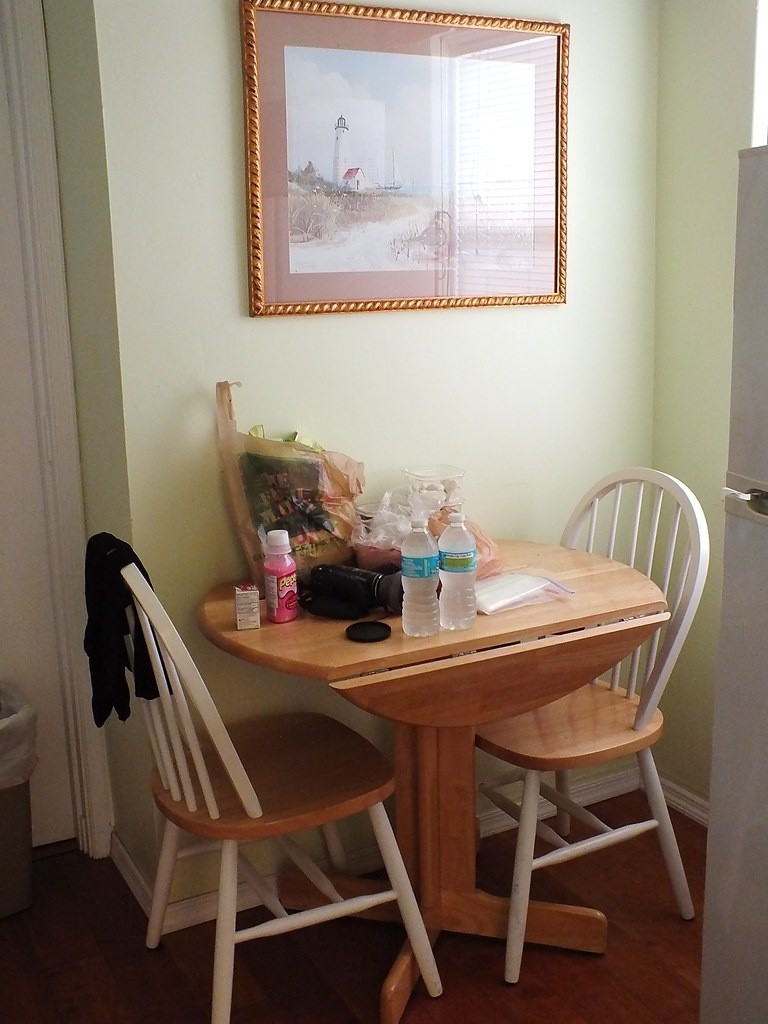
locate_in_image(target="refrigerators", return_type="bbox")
[703,146,768,1024]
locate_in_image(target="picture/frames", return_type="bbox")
[240,0,571,318]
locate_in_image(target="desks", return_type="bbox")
[195,538,670,1024]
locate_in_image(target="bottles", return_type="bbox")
[438,513,476,630]
[400,519,439,637]
[264,530,298,623]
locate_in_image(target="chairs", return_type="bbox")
[87,533,443,1024]
[476,466,710,986]
